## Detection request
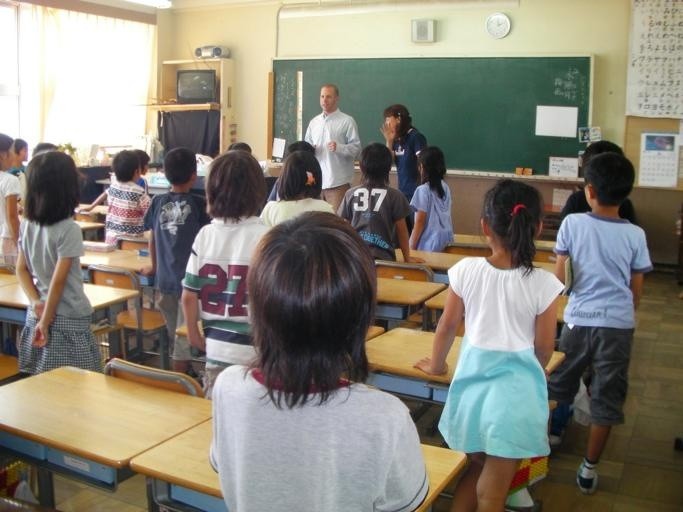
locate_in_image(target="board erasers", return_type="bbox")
[515,167,532,176]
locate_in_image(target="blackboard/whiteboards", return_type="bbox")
[271,54,595,183]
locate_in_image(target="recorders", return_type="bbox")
[195,45,230,59]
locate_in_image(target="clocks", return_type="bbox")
[486,12,511,38]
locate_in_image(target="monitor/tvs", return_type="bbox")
[176,69,217,104]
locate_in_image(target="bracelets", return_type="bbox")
[32,300,41,310]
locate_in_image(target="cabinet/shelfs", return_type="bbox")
[147,58,236,158]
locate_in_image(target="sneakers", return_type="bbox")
[576,462,600,495]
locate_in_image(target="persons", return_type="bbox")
[207,211,429,512]
[1,135,328,267]
[141,145,212,374]
[581,131,589,140]
[337,142,426,264]
[409,146,456,253]
[545,152,657,495]
[304,85,362,214]
[181,150,276,398]
[559,139,636,222]
[380,105,426,234]
[16,149,106,378]
[413,177,566,512]
